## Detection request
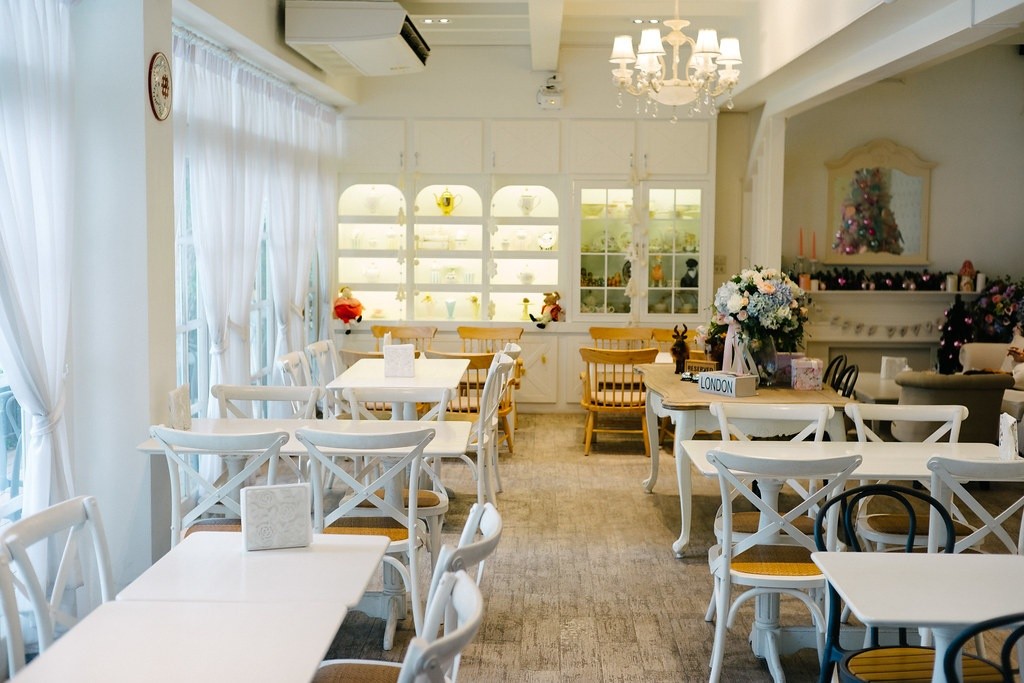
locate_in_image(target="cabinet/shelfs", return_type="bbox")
[570,175,714,322]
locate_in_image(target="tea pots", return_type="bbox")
[434,188,462,216]
[514,188,541,216]
[582,303,614,314]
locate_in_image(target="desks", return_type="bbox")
[7,601,348,681]
[669,440,1019,658]
[115,532,392,610]
[325,357,472,487]
[811,552,1023,659]
[633,361,849,558]
[137,414,474,554]
[839,363,1020,432]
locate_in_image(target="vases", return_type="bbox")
[733,324,778,385]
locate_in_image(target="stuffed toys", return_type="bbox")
[529,292,565,329]
[333,287,365,335]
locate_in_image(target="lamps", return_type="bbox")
[609,0,742,127]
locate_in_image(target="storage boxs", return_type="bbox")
[791,355,822,391]
[775,351,807,381]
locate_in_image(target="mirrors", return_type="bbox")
[816,139,941,268]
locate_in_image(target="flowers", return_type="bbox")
[716,266,810,344]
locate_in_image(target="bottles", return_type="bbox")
[585,291,595,307]
[607,200,632,217]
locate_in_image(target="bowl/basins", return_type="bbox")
[582,203,604,218]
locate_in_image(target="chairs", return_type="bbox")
[580,288,1023,682]
[0,312,530,682]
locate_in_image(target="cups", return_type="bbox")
[684,234,696,252]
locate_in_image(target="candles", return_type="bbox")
[812,229,818,259]
[798,226,804,256]
